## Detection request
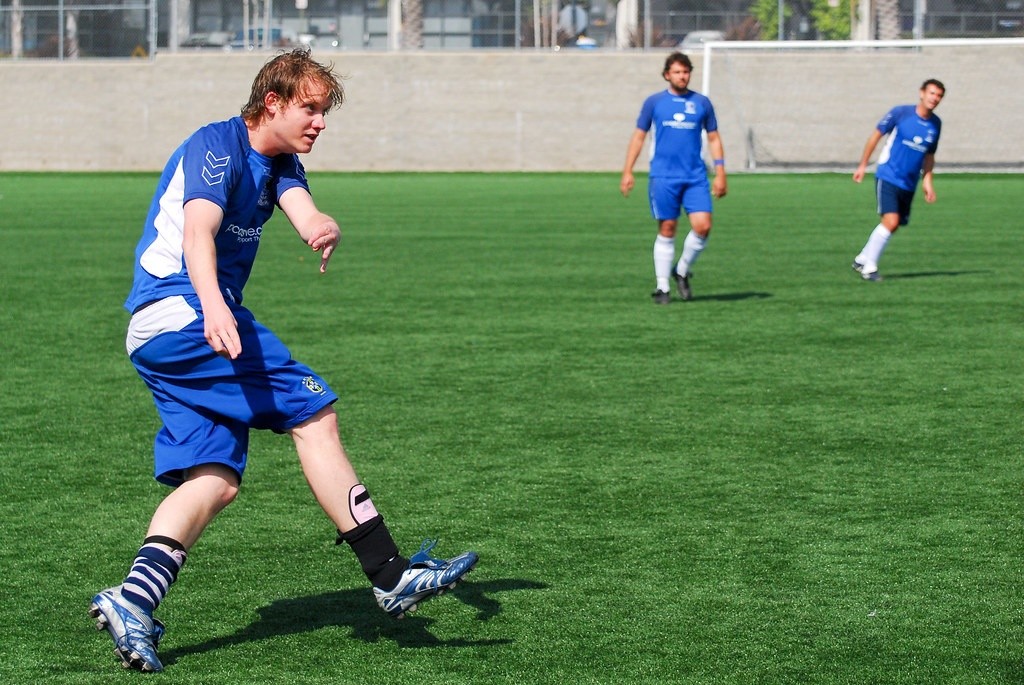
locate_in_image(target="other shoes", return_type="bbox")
[861,269,882,282]
[851,260,864,271]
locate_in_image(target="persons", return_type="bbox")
[88,48,480,674]
[851,78,946,282]
[619,53,728,304]
[559,0,587,42]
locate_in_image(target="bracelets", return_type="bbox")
[714,159,724,165]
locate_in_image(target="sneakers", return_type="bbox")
[373,536,479,619]
[651,289,669,305]
[88,584,166,674]
[671,263,691,300]
